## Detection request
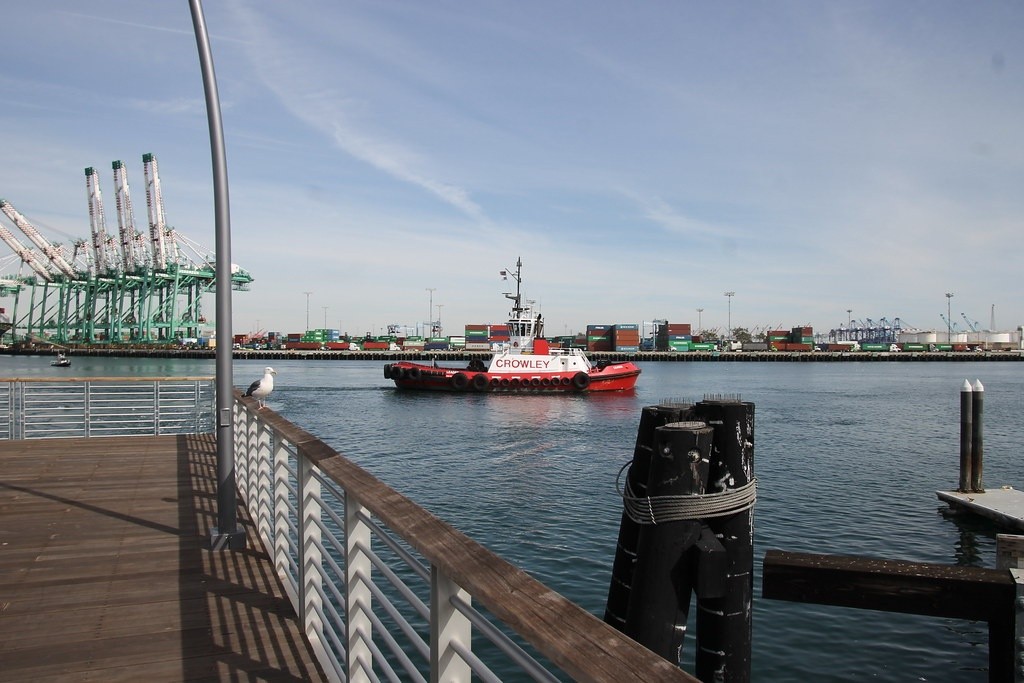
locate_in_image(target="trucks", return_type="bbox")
[861,343,901,352]
[901,344,982,351]
[325,342,400,351]
[741,345,777,353]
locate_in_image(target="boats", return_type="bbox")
[384,257,642,393]
[51,350,71,366]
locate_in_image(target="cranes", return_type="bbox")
[940,312,981,332]
[0,153,255,348]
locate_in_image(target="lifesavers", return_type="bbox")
[502,377,511,386]
[511,378,519,385]
[574,372,590,389]
[452,373,467,389]
[471,373,489,390]
[542,377,551,386]
[522,378,530,387]
[409,367,420,379]
[513,341,520,347]
[492,378,500,386]
[531,378,540,387]
[552,377,560,386]
[391,367,401,378]
[562,377,571,386]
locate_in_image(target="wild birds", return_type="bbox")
[240,366,277,411]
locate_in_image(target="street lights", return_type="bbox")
[696,307,704,331]
[435,303,446,337]
[723,290,736,340]
[945,292,954,343]
[847,310,853,340]
[303,291,313,331]
[425,288,437,341]
[320,306,329,328]
